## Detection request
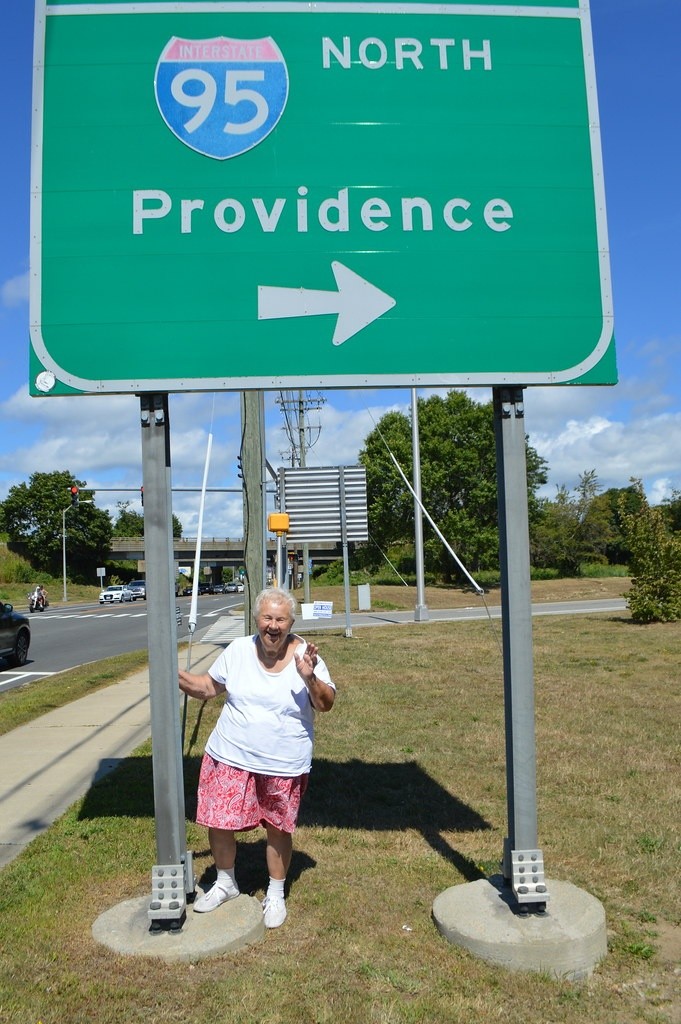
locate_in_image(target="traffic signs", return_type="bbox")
[32,1,619,395]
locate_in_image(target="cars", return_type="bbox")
[182,583,244,596]
[99,585,133,604]
[127,579,146,601]
[0,600,31,674]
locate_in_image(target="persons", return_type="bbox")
[178,585,336,928]
[39,585,48,605]
[36,587,43,607]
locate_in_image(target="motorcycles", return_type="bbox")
[29,598,50,612]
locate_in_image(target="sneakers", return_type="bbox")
[192,880,239,913]
[260,895,286,929]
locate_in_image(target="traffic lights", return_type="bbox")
[69,484,79,507]
[140,485,143,505]
